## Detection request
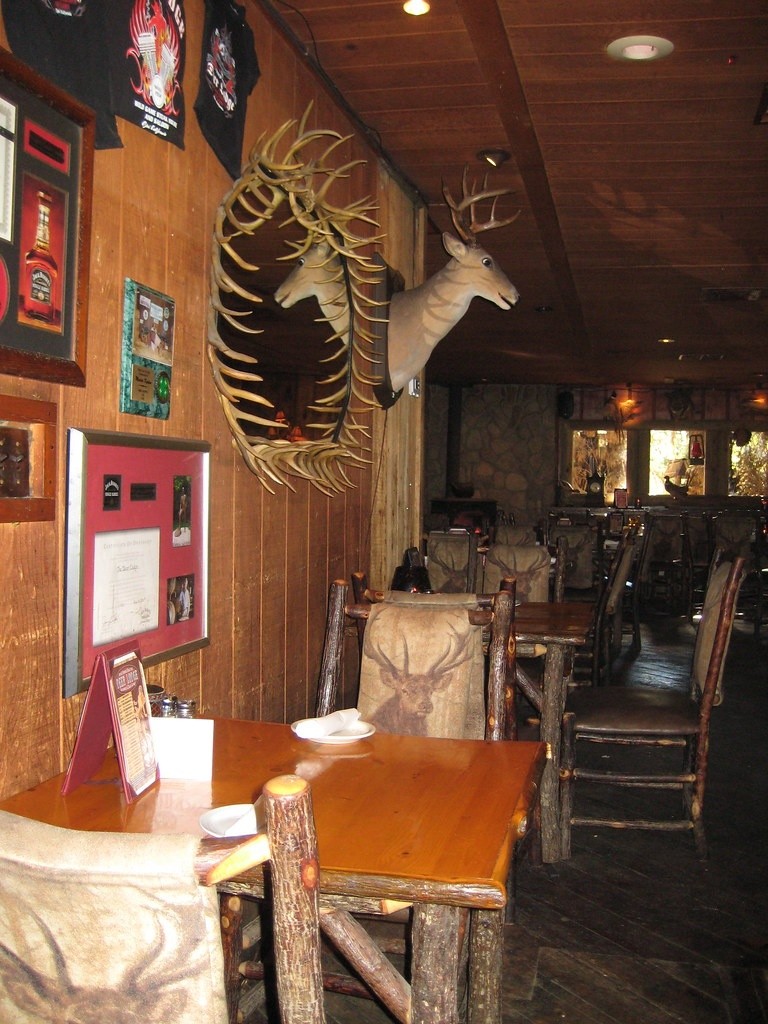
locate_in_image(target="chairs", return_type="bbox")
[0,498,768,1024]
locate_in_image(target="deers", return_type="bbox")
[388,160,522,393]
[272,191,350,347]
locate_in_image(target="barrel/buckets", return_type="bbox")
[147,683,165,717]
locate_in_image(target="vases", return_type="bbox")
[453,478,475,499]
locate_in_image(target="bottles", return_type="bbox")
[178,699,194,718]
[161,699,175,717]
[25,190,58,320]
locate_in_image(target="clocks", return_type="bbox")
[585,467,606,507]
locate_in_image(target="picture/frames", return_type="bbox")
[0,46,98,388]
[120,274,175,422]
[63,427,211,700]
[614,487,628,509]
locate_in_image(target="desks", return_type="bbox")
[431,590,598,864]
[427,496,498,529]
[0,713,547,1024]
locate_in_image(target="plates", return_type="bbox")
[290,717,376,744]
[200,805,264,838]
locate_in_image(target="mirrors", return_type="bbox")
[214,166,359,457]
